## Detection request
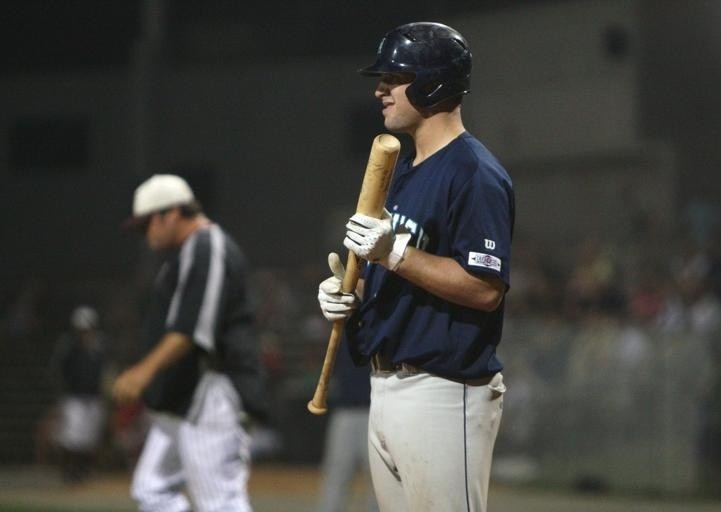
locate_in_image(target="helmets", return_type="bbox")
[356,22,477,115]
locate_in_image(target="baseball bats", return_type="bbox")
[307,134,401,416]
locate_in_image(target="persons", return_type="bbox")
[110,173,266,511]
[49,207,720,498]
[318,20,514,509]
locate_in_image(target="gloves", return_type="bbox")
[315,251,362,325]
[341,211,416,275]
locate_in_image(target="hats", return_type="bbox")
[116,172,198,232]
